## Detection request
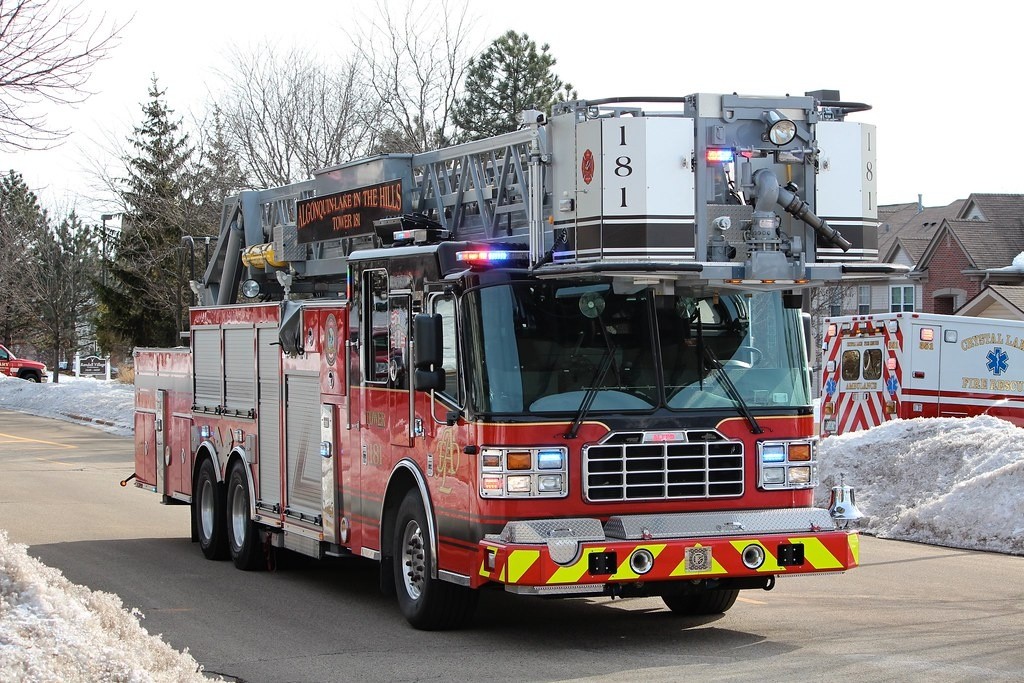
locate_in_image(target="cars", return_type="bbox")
[59,362,76,370]
[0,343,49,385]
[111,368,120,378]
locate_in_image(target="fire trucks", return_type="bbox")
[117,87,914,632]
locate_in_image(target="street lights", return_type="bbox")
[101,214,113,296]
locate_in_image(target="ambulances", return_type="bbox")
[818,311,1024,442]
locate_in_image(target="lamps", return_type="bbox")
[762,110,797,146]
